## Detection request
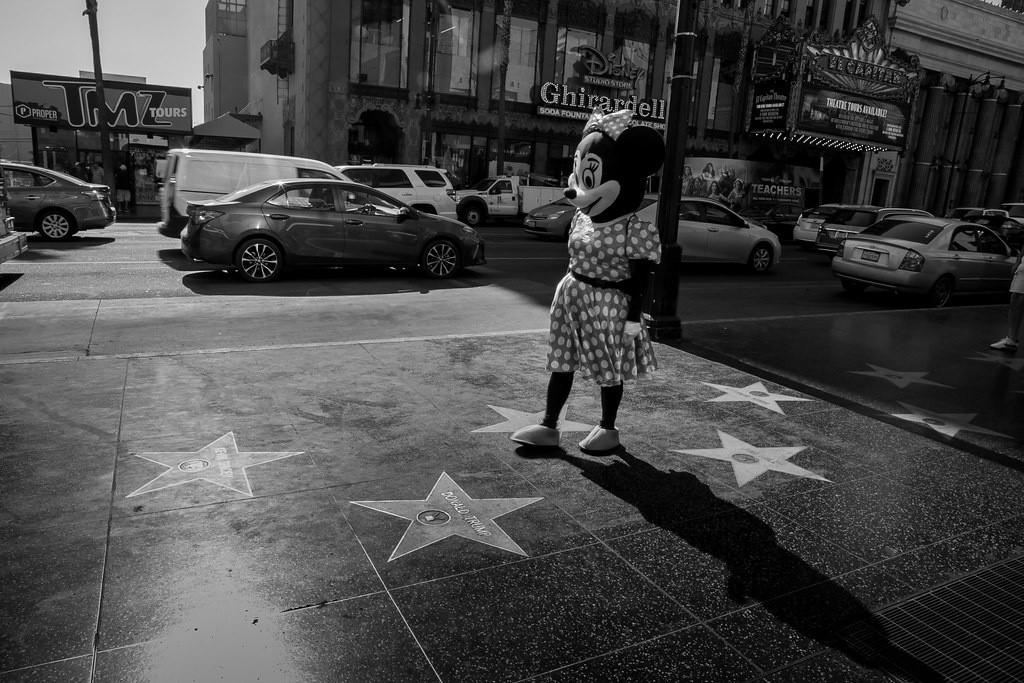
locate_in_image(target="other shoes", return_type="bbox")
[125,208,130,212]
[509,424,559,445]
[579,425,619,450]
[118,208,123,212]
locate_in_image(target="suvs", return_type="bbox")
[792,202,882,249]
[815,207,935,258]
[0,176,29,265]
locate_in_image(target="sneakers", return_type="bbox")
[989,336,1019,351]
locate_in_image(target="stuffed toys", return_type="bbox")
[512,107,664,452]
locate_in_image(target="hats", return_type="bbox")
[120,165,126,170]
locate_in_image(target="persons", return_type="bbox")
[683,162,746,211]
[115,164,131,213]
[989,248,1024,350]
[90,161,104,184]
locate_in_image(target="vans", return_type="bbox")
[155,146,404,239]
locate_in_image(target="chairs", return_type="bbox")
[320,190,336,211]
[308,188,323,211]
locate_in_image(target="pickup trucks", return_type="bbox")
[453,174,577,228]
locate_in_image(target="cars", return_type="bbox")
[180,178,489,284]
[325,163,459,223]
[830,217,1024,307]
[634,194,783,277]
[942,207,1009,223]
[0,161,117,242]
[521,191,579,241]
[1000,202,1024,226]
